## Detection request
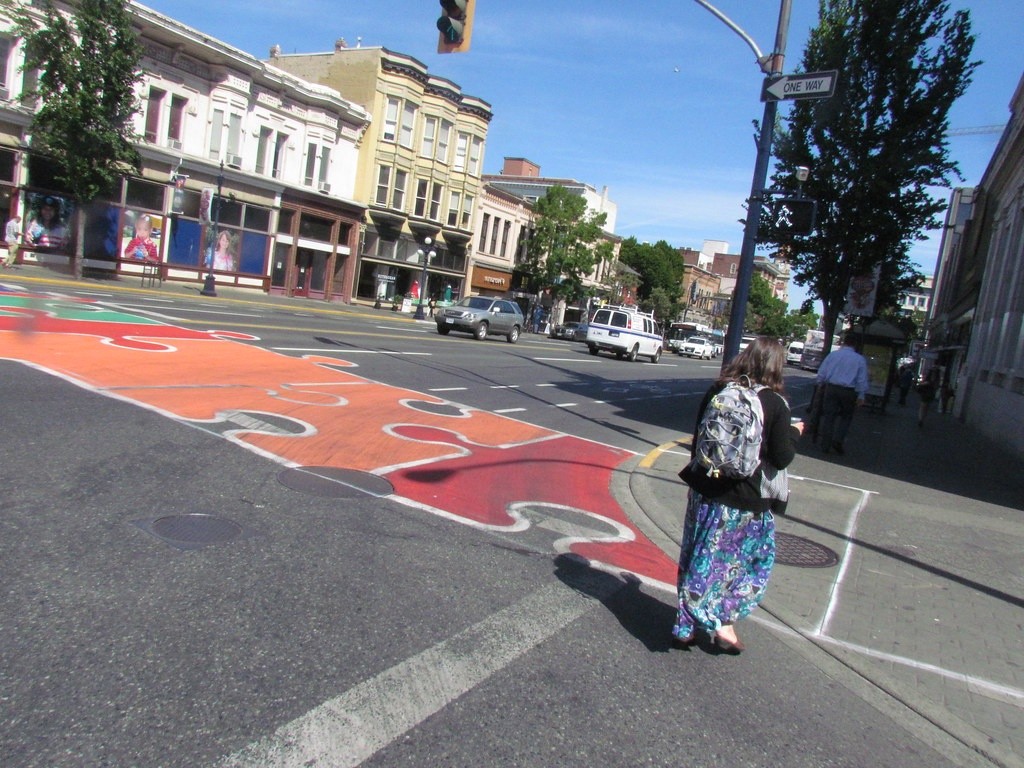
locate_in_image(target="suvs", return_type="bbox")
[434,293,525,344]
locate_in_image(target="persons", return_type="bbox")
[672,338,804,650]
[917,378,934,426]
[818,335,868,452]
[25,196,68,249]
[124,215,156,262]
[0,215,24,269]
[207,231,233,271]
[897,362,916,406]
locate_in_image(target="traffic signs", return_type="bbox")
[759,70,839,103]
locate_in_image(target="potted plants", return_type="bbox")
[393,295,401,310]
[374,295,385,308]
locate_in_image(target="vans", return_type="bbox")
[786,340,804,365]
[584,299,664,364]
[739,336,757,354]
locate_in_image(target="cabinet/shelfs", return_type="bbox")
[141,260,163,288]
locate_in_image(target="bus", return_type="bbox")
[798,329,843,371]
[664,322,726,354]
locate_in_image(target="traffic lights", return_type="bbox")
[771,196,818,236]
[434,0,476,54]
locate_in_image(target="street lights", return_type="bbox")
[412,236,439,321]
[199,172,228,297]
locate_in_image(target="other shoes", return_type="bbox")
[831,440,844,454]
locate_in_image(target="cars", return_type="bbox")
[677,336,714,360]
[550,321,588,342]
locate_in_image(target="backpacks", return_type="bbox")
[696,373,773,479]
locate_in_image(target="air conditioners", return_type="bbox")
[167,139,182,150]
[318,182,331,192]
[225,154,242,166]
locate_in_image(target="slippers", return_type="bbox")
[716,635,742,653]
[674,634,694,649]
